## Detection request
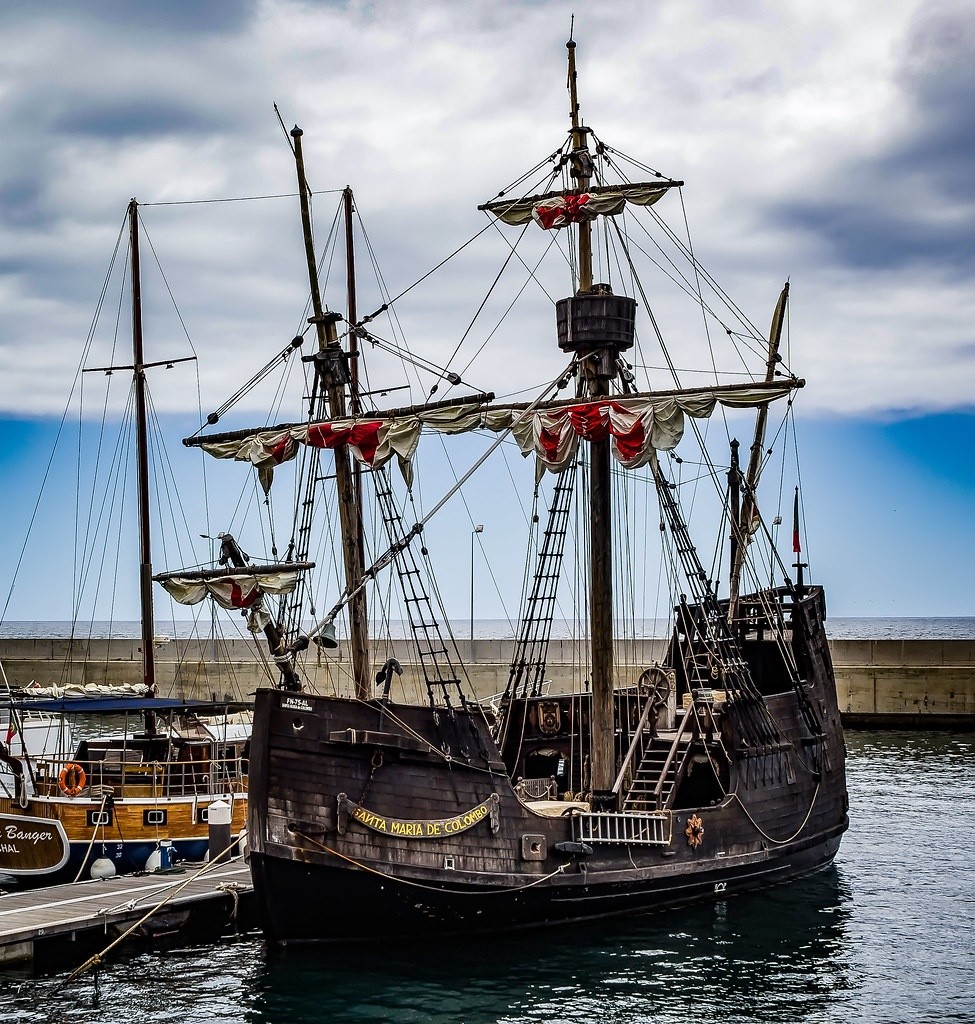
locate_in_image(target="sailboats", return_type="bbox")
[0,183,483,888]
[151,7,854,949]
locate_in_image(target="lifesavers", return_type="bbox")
[59,763,86,795]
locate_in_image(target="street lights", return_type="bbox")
[199,531,226,661]
[770,516,783,589]
[469,525,485,666]
